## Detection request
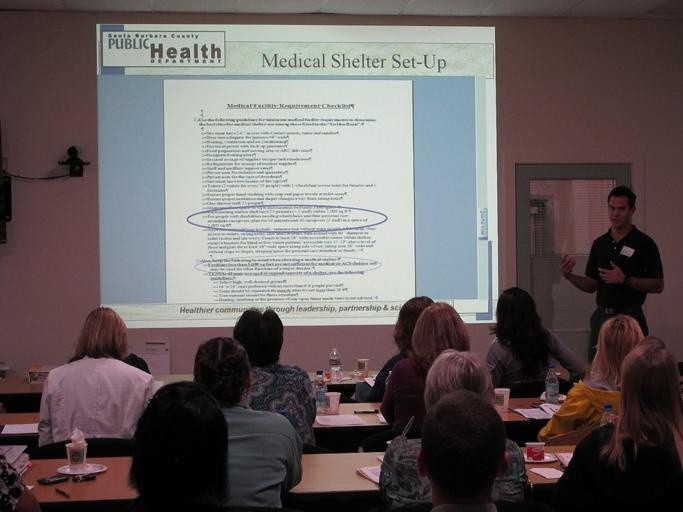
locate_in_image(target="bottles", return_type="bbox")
[328,345,343,367]
[601,404,616,427]
[312,369,326,415]
[544,363,559,404]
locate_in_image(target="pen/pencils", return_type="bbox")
[560,463,566,469]
[55,487,71,497]
[354,409,379,413]
[527,401,538,408]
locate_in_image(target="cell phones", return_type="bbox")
[37,474,68,484]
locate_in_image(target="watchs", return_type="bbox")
[623,275,631,286]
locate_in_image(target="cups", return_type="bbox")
[357,358,369,375]
[65,443,88,470]
[524,441,546,461]
[493,388,511,413]
[325,391,341,414]
[329,366,341,383]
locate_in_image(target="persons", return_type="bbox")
[537,314,645,443]
[380,302,470,435]
[0,455,41,512]
[487,287,554,398]
[416,389,511,512]
[38,307,163,447]
[379,348,528,510]
[233,310,317,453]
[127,380,230,512]
[552,336,683,512]
[559,185,664,365]
[367,296,433,402]
[192,336,304,512]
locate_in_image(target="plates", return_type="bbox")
[58,461,109,477]
[523,453,558,462]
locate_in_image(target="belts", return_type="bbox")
[604,308,613,314]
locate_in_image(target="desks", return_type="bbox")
[0,441,578,512]
[0,369,380,407]
[0,394,565,441]
